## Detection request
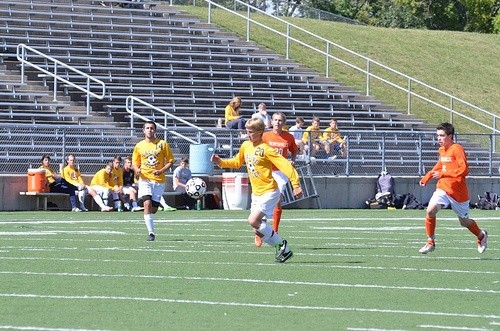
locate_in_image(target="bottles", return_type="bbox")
[197,198,201,210]
[118,201,122,212]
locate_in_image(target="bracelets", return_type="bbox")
[160,169,163,173]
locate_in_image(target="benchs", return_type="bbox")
[18,191,221,211]
[0,0,500,173]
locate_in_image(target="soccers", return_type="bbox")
[185,176,206,198]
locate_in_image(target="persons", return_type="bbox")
[173,157,192,211]
[255,111,298,248]
[252,102,272,130]
[419,122,488,254]
[210,117,303,263]
[288,117,348,162]
[224,97,250,140]
[37,155,177,211]
[131,121,175,242]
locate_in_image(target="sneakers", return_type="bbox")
[419,242,435,254]
[275,240,287,259]
[148,233,155,241]
[477,230,488,254]
[275,251,292,263]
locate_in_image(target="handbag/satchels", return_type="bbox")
[392,193,405,207]
[365,197,387,209]
[481,191,499,209]
[401,193,421,209]
[377,173,394,194]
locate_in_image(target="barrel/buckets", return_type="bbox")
[189,144,214,175]
[27,169,46,192]
[222,172,249,210]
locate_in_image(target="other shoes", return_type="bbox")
[158,207,163,210]
[72,208,82,212]
[255,235,262,247]
[118,208,123,211]
[131,208,134,212]
[164,206,176,211]
[133,206,144,211]
[80,206,88,212]
